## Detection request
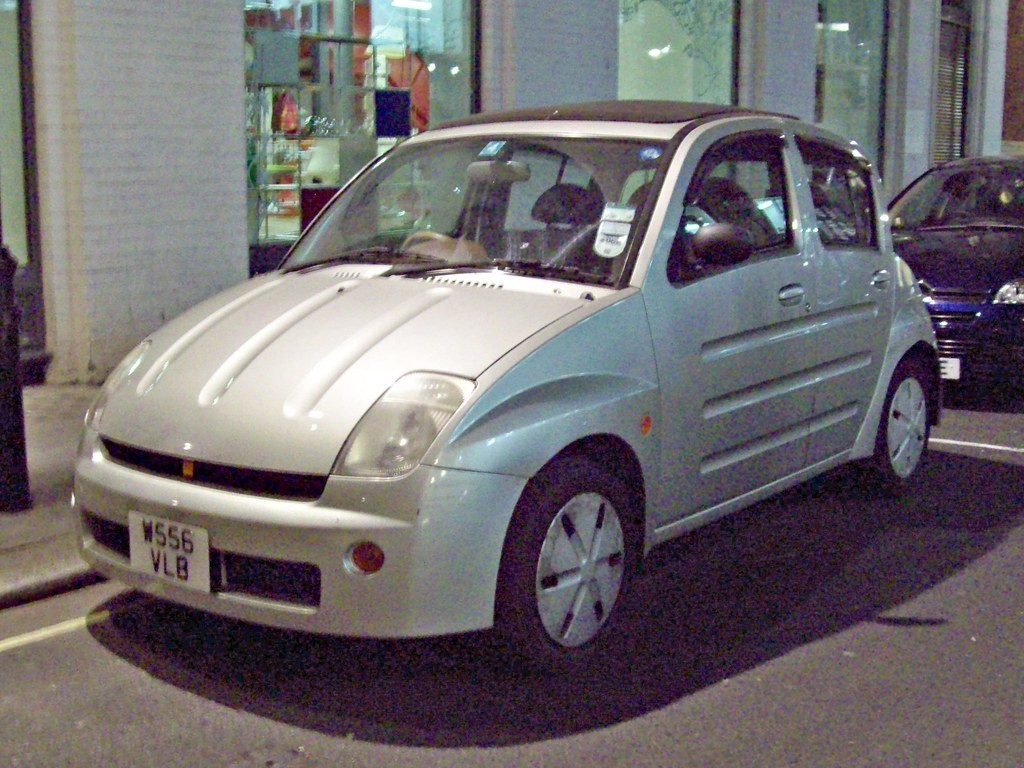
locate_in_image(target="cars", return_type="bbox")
[885,154,1024,390]
[70,101,943,676]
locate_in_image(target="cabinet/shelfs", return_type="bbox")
[252,30,414,239]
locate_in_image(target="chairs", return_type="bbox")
[767,183,832,243]
[611,177,761,278]
[958,182,1021,225]
[505,182,604,273]
